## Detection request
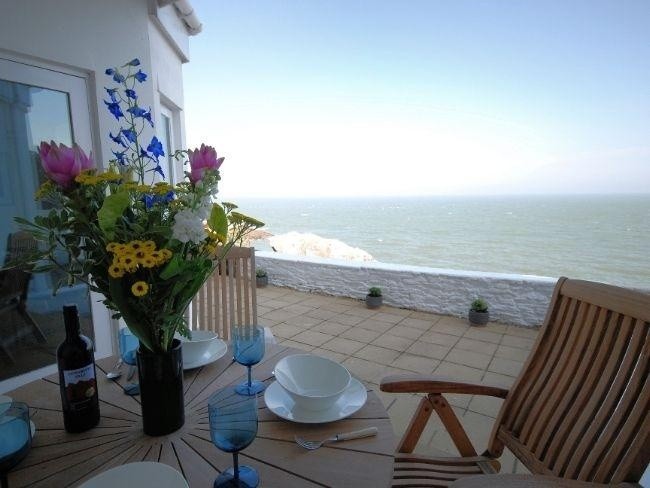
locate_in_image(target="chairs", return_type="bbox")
[379,276,650,488]
[0,228,44,367]
[185,245,259,342]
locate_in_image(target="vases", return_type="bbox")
[135,347,183,438]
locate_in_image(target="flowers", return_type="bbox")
[27,52,257,346]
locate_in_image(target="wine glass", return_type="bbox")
[1,402,29,488]
[119,325,140,396]
[231,324,267,395]
[207,385,261,488]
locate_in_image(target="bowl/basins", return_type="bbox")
[275,356,351,410]
[0,395,12,417]
[174,330,218,360]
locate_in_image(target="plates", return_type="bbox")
[76,462,189,488]
[183,340,227,369]
[263,377,367,424]
[1,416,36,457]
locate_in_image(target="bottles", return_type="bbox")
[57,303,100,432]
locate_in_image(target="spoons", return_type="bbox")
[107,359,121,379]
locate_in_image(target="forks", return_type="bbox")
[294,427,380,451]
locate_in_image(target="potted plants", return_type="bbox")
[364,287,383,310]
[255,268,268,288]
[468,297,490,325]
